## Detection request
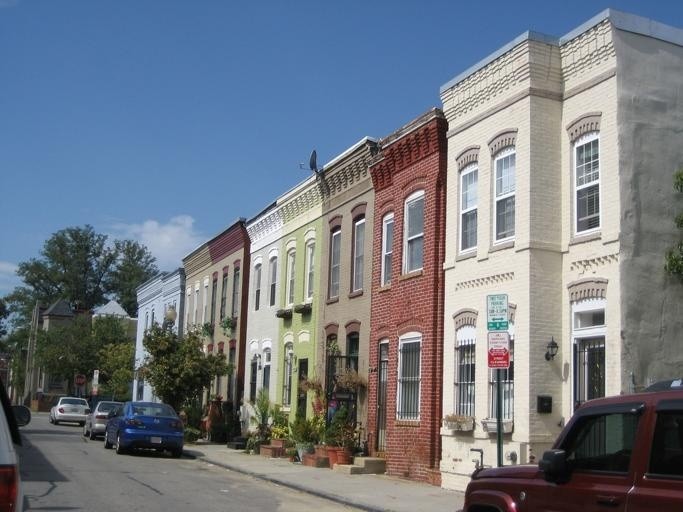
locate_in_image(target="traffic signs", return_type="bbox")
[487,295,508,331]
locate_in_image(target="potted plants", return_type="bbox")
[270,407,362,465]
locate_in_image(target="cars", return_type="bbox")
[104,400,184,457]
[83,400,123,440]
[0,378,32,511]
[48,396,89,425]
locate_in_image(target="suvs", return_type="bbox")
[461,379,682,511]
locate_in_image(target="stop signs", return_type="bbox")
[74,374,85,386]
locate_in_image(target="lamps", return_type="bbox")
[545,336,558,361]
